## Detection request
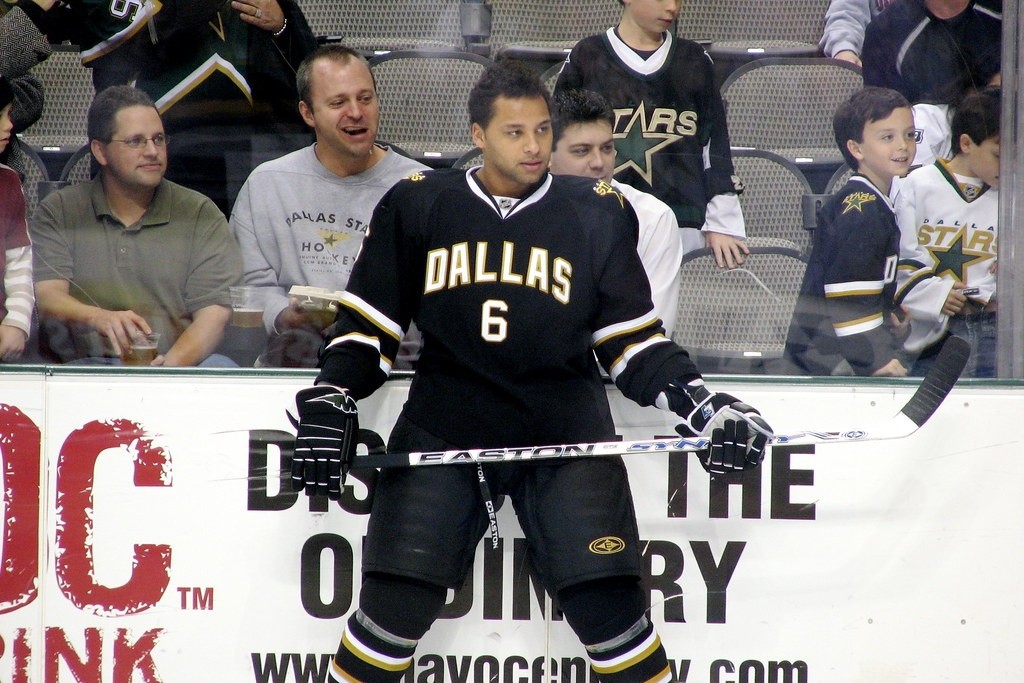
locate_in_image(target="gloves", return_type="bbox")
[656,378,774,484]
[290,386,359,500]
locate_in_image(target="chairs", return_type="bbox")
[9,0,864,358]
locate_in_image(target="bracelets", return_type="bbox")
[273,18,287,36]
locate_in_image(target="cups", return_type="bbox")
[229,285,268,328]
[121,331,161,367]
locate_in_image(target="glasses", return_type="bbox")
[110,133,172,149]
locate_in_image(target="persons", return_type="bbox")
[230,45,437,376]
[0,1,320,368]
[548,87,682,376]
[784,0,1005,376]
[549,0,750,271]
[290,61,776,683]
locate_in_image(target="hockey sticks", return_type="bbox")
[349,335,972,468]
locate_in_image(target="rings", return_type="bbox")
[254,8,262,17]
